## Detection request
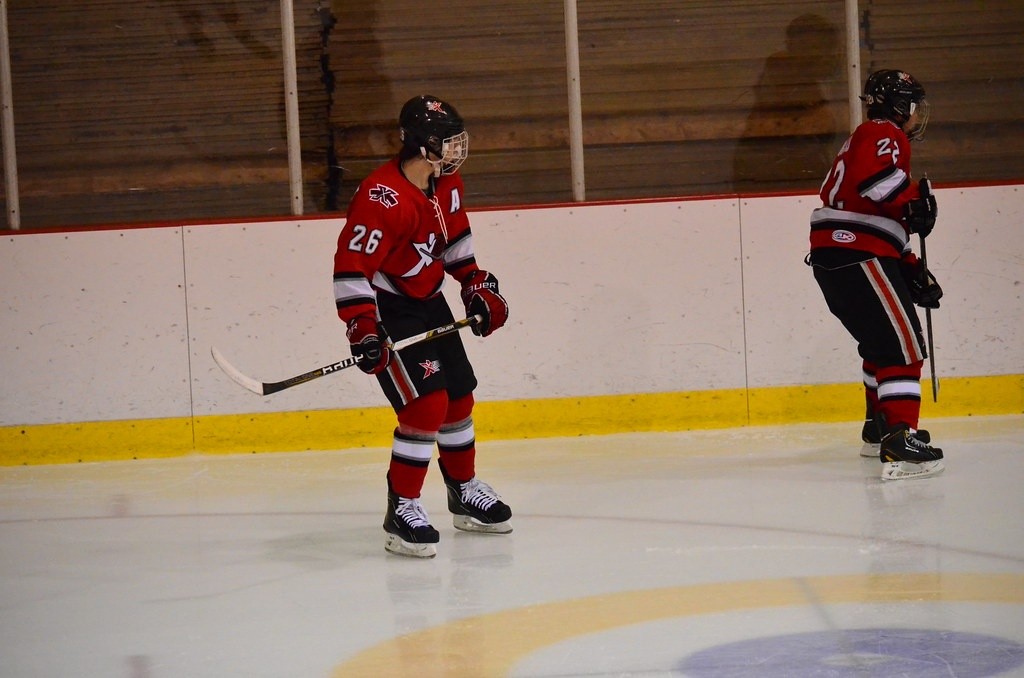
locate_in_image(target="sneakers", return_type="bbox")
[437,458,513,533]
[860,397,933,457]
[383,471,440,558]
[876,412,945,482]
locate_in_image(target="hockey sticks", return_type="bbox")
[210,314,482,397]
[918,174,941,402]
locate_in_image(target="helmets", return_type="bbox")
[862,70,928,140]
[398,95,464,175]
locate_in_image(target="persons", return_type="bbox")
[334,96,513,544]
[809,68,946,464]
[735,13,839,191]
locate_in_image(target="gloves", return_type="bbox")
[905,178,938,237]
[461,270,509,336]
[345,315,394,374]
[903,258,943,309]
